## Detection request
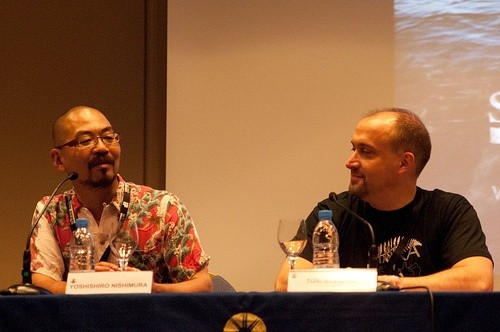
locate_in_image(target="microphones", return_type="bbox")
[328,191,377,268]
[21,172,79,285]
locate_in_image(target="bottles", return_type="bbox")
[311,210,340,268]
[70,218,95,272]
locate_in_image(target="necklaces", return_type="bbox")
[65,179,129,261]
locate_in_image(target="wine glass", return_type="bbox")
[277,218,307,270]
[108,218,138,272]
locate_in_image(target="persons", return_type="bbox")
[274,107,495,293]
[30,106,213,295]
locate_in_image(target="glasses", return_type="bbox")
[56,131,121,149]
